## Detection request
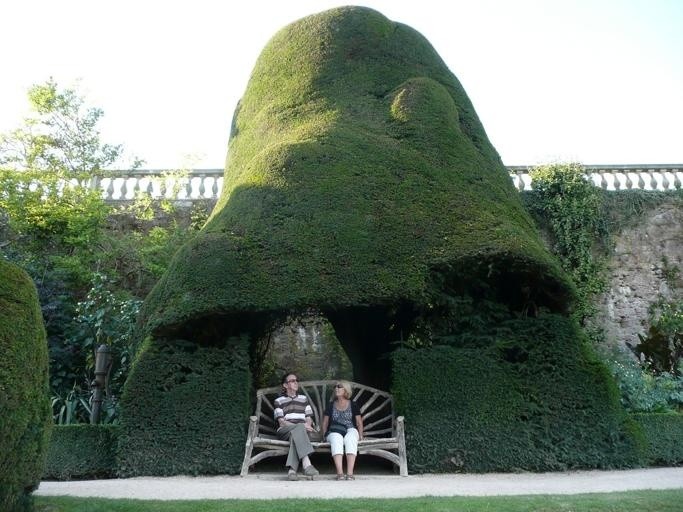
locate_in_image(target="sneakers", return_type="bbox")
[287,470,297,481]
[337,474,353,480]
[303,465,319,476]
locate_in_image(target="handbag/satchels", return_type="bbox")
[329,421,346,436]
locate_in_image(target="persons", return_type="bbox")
[323,379,364,481]
[272,372,320,481]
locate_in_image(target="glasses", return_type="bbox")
[334,385,343,388]
[286,379,299,383]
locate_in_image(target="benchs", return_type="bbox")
[239,379,411,479]
